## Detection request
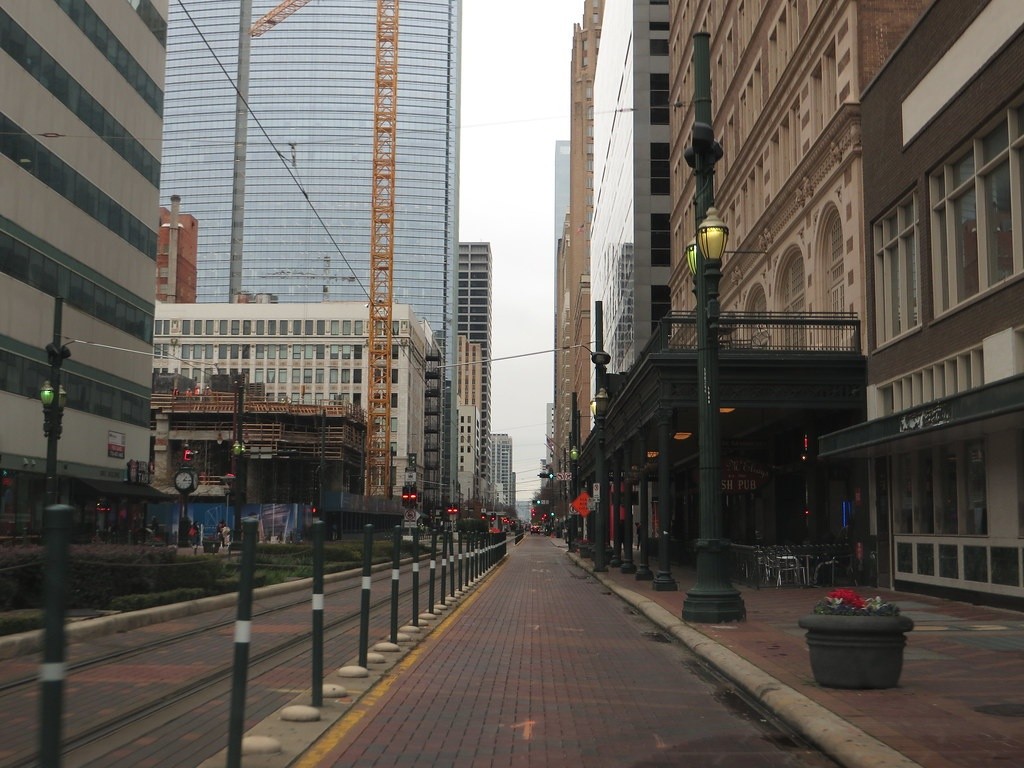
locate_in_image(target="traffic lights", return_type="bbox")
[540,474,554,478]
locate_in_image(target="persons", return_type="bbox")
[418,523,431,540]
[153,517,230,554]
[620,519,640,553]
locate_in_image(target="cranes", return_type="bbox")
[248,0,399,498]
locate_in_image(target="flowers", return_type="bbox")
[812,588,902,613]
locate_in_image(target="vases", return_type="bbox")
[801,608,915,690]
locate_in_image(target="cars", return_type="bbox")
[531,525,541,535]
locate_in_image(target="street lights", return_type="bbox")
[568,391,580,552]
[41,295,72,508]
[232,386,247,542]
[589,300,612,573]
[682,31,749,625]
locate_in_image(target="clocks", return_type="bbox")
[193,472,198,490]
[175,471,193,489]
